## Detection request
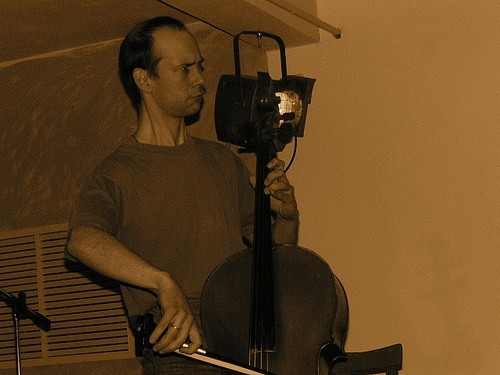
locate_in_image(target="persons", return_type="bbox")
[66,15,299,375]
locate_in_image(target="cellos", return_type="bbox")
[198,148,350,375]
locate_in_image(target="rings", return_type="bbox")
[170,323,179,331]
